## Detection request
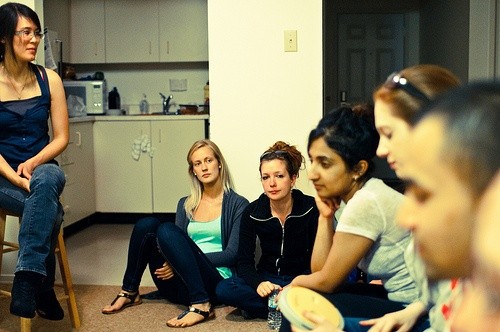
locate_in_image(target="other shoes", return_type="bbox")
[9,269,48,318]
[240,308,255,320]
[36,288,64,321]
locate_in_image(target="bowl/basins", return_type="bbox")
[107,109,122,116]
[179,104,197,114]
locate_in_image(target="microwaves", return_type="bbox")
[59,79,108,115]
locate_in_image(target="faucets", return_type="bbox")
[152,92,178,115]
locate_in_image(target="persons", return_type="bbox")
[0,2,69,321]
[101,140,249,328]
[281,103,414,332]
[216,141,319,319]
[291,64,500,332]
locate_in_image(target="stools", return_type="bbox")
[0,208,80,332]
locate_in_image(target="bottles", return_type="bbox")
[267,284,283,332]
[109,87,121,110]
[202,81,209,114]
[64,66,76,80]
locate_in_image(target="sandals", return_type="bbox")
[102,291,142,314]
[166,304,215,328]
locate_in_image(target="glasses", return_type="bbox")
[15,30,41,40]
[385,71,432,109]
[259,150,296,164]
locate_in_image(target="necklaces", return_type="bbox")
[4,73,30,100]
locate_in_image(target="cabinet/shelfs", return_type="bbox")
[61,120,211,241]
[43,0,208,64]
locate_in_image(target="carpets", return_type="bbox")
[0,282,282,332]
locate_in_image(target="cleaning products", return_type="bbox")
[138,92,149,114]
